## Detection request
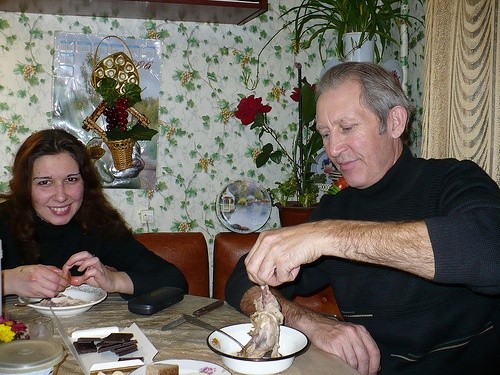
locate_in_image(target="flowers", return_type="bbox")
[0,312,31,342]
[235,77,331,208]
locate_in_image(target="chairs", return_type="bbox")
[214,231,345,323]
[132,233,211,299]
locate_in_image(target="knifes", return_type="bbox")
[161,300,224,332]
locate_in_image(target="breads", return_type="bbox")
[144,364,179,375]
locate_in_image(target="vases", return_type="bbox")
[276,202,319,227]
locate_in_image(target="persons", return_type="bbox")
[225,61,500,375]
[0,128,189,300]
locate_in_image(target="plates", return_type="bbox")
[129,359,232,374]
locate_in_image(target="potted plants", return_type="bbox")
[278,0,426,63]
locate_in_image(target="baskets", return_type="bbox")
[106,138,134,170]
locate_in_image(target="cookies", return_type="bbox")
[73,332,143,364]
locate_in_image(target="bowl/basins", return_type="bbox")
[19,283,107,318]
[0,339,67,375]
[207,322,310,375]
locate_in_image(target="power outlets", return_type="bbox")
[141,210,154,224]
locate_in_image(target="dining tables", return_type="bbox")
[0,292,361,375]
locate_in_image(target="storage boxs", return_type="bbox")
[50,305,159,375]
